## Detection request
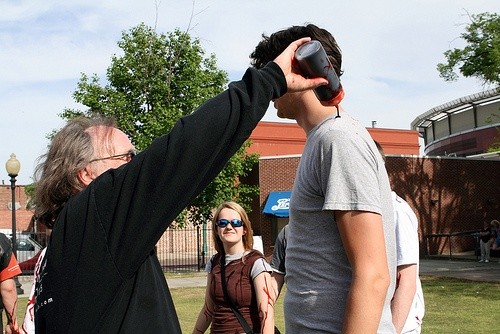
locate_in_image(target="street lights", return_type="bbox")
[5,152,21,261]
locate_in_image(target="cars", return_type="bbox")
[0,228,42,271]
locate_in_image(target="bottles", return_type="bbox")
[295,41,345,108]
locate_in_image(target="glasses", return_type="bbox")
[216,219,243,228]
[88,149,136,163]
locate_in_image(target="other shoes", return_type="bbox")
[479,260,484,263]
[485,260,489,263]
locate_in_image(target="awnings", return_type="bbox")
[262,191,293,217]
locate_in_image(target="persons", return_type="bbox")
[191,202,275,334]
[0,232,23,334]
[24,37,329,334]
[249,23,426,334]
